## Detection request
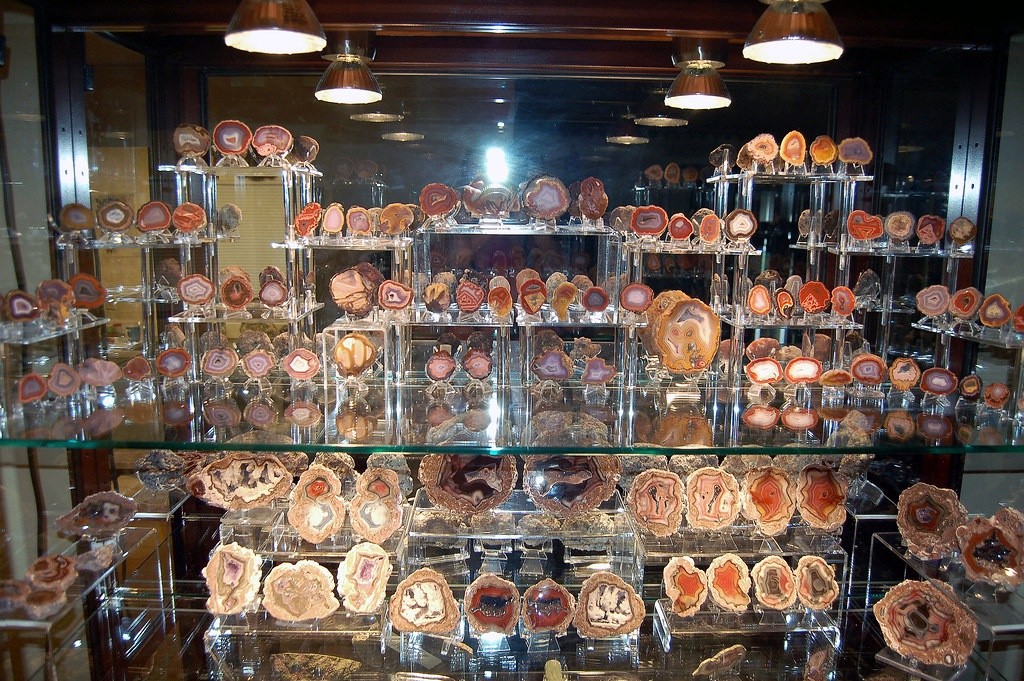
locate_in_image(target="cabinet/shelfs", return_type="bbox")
[317,153,392,211]
[634,163,714,277]
[1,120,1024,681]
[882,173,948,217]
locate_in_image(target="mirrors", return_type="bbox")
[71,62,976,678]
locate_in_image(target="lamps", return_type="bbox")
[742,0,844,64]
[634,88,689,127]
[381,127,425,142]
[313,53,382,105]
[349,84,405,123]
[605,114,649,145]
[223,0,327,55]
[664,59,731,110]
